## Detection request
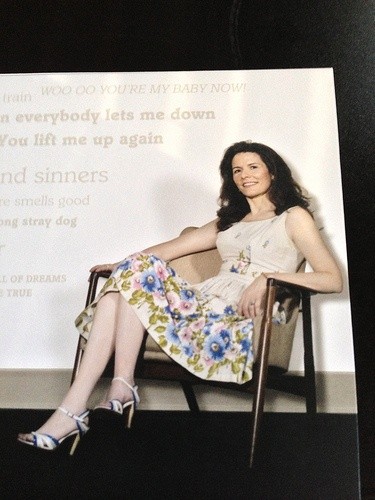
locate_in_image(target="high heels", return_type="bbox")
[17,406,90,455]
[93,377,141,429]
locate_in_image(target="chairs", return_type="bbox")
[68,229,318,472]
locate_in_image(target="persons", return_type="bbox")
[16,140,343,456]
